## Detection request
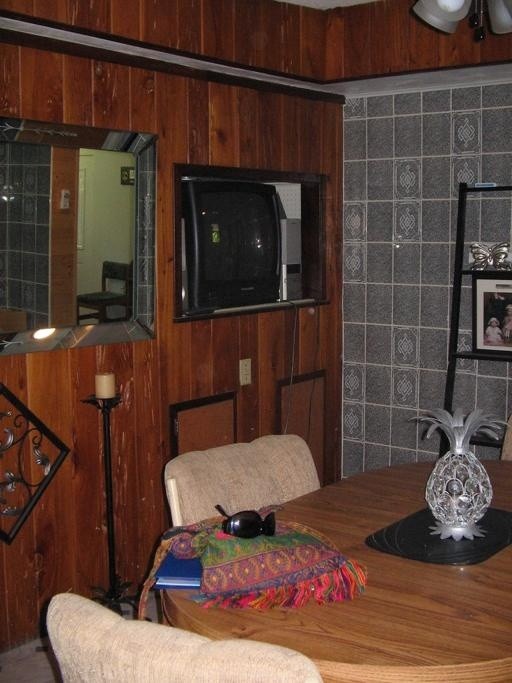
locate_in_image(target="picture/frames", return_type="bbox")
[472,274,512,355]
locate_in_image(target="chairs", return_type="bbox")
[46,592,325,683]
[164,433,320,527]
[74,261,129,325]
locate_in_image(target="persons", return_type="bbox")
[485,304,512,342]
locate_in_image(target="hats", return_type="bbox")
[505,304,512,310]
[488,317,499,325]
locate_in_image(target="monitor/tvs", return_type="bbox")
[181,182,282,310]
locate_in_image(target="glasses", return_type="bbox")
[215,504,275,539]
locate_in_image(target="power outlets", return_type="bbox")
[238,358,252,386]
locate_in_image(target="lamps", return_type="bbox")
[411,0,512,42]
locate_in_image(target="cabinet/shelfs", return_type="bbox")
[441,181,512,461]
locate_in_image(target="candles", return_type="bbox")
[94,371,115,400]
